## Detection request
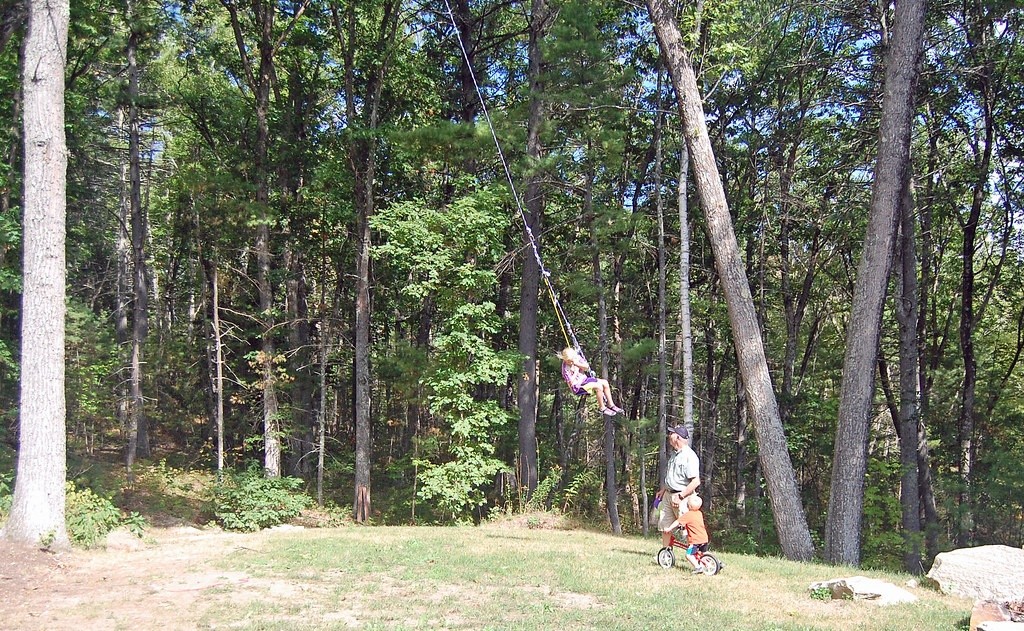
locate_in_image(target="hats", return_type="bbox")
[668,424,689,439]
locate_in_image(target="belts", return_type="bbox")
[667,489,681,493]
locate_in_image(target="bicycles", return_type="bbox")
[658,526,720,576]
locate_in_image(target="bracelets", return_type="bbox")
[679,494,684,500]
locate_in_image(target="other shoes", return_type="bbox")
[692,566,703,573]
[651,555,658,565]
[606,405,624,414]
[598,407,616,417]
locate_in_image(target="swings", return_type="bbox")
[444,0,598,396]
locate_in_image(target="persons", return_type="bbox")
[652,424,700,564]
[662,495,726,575]
[556,348,626,416]
